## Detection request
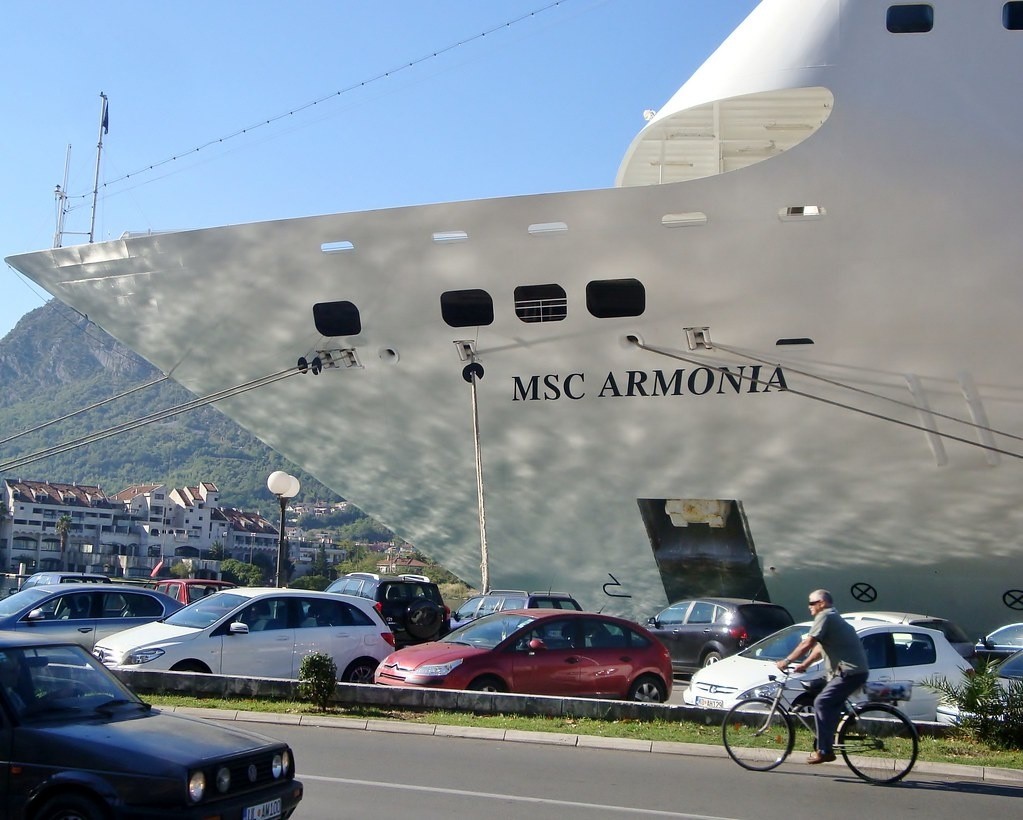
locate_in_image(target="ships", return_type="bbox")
[0,1,1022,641]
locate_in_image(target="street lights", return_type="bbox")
[266,469,301,619]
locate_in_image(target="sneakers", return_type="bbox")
[807,749,837,764]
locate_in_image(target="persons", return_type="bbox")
[776,590,869,763]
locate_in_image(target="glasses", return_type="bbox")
[809,600,820,605]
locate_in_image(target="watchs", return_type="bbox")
[785,658,792,664]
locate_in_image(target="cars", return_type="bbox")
[839,611,986,674]
[154,578,237,606]
[8,571,111,595]
[644,599,794,675]
[973,623,1023,663]
[0,629,304,820]
[374,608,674,705]
[92,586,396,686]
[934,649,1023,739]
[683,620,977,723]
[0,582,186,659]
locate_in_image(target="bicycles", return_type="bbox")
[721,661,922,785]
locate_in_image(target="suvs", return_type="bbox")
[447,587,583,639]
[323,571,451,648]
